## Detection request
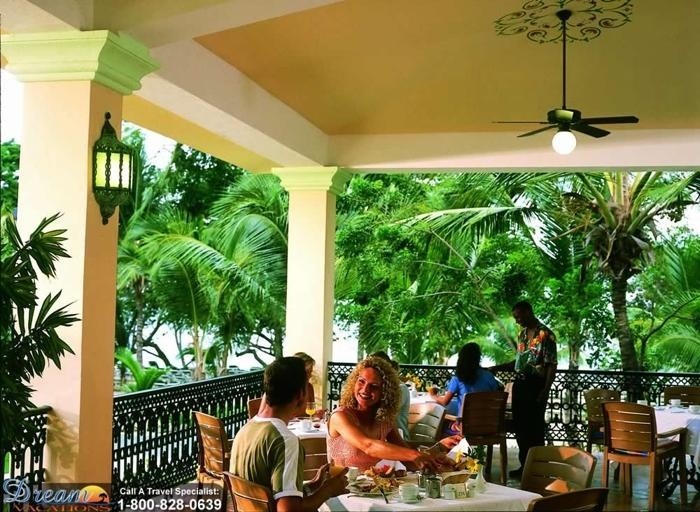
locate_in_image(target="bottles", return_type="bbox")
[426,477,442,498]
[418,473,430,489]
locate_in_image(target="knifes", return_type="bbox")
[378,486,389,504]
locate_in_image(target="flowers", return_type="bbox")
[453,443,488,476]
[403,373,422,389]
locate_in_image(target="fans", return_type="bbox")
[490,9,639,137]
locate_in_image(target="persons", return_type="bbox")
[428,342,501,438]
[228,356,351,510]
[255,351,316,418]
[367,350,412,444]
[325,356,463,474]
[488,300,559,480]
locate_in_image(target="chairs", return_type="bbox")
[220,470,280,511]
[247,397,266,423]
[662,384,700,409]
[599,401,689,511]
[581,390,632,492]
[185,410,234,512]
[517,446,598,502]
[528,487,612,512]
[442,391,510,486]
[501,379,527,439]
[296,435,328,485]
[401,404,447,455]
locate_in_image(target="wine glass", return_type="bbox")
[425,381,433,393]
[305,402,316,432]
[328,456,345,478]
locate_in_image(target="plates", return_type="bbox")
[688,410,700,414]
[666,405,684,408]
[346,480,399,495]
[392,493,424,503]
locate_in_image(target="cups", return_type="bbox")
[669,399,681,406]
[637,400,648,406]
[399,482,419,500]
[424,479,443,498]
[688,405,700,413]
[345,466,359,481]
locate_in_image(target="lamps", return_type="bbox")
[93,109,138,226]
[550,129,576,155]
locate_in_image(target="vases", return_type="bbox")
[474,464,490,494]
[411,382,418,397]
